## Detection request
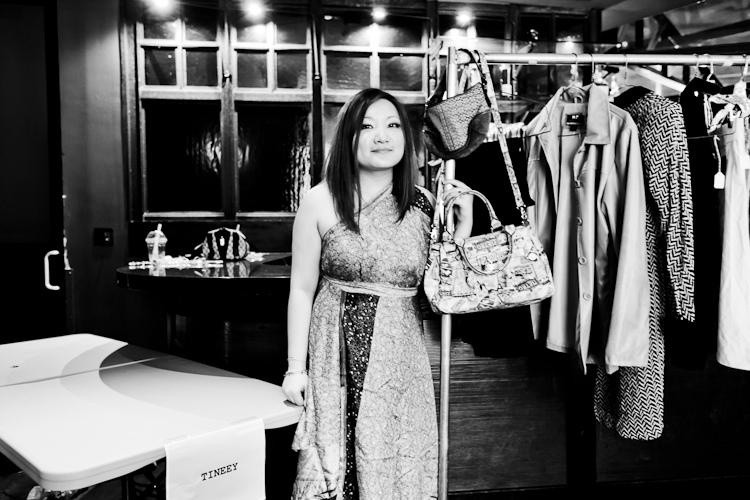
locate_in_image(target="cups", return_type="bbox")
[145,230,168,269]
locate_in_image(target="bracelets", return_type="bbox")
[282,366,310,377]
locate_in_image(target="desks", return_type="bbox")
[117,253,291,371]
[0,333,305,499]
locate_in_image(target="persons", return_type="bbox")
[278,86,475,500]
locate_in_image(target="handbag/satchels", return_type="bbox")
[424,219,554,314]
[421,82,492,159]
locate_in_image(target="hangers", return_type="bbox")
[494,52,750,142]
